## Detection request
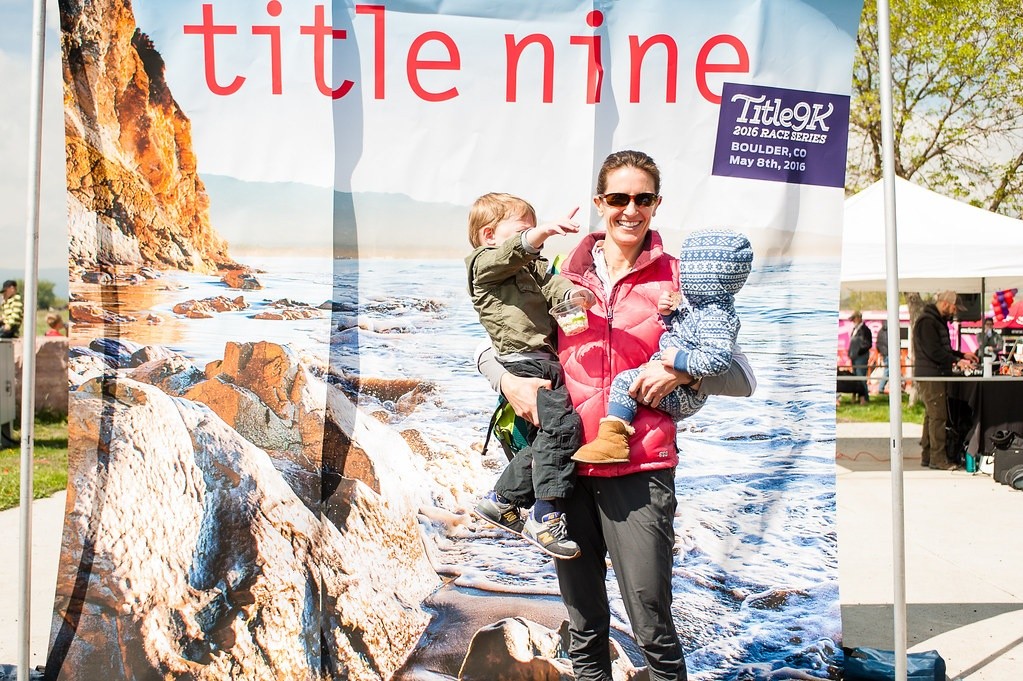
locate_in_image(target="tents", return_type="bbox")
[840,171,1023,296]
[956,301,1023,352]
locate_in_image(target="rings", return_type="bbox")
[644,397,653,403]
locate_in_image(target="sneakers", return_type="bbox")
[520,506,581,560]
[474,490,530,537]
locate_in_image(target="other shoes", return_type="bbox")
[921,458,930,466]
[929,462,957,470]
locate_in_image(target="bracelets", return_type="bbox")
[687,375,702,390]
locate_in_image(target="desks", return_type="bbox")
[837,375,1022,476]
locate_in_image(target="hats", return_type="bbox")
[849,312,862,322]
[938,290,968,312]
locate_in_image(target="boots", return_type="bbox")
[570,417,635,463]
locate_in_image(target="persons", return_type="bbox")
[847,311,873,405]
[462,191,596,560]
[476,152,759,681]
[572,228,752,462]
[976,317,1004,372]
[0,280,24,338]
[913,292,980,470]
[45,313,68,337]
[875,319,889,395]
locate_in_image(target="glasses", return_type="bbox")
[598,192,659,208]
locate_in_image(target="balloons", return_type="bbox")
[989,288,1018,321]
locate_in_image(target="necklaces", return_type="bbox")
[606,263,634,273]
